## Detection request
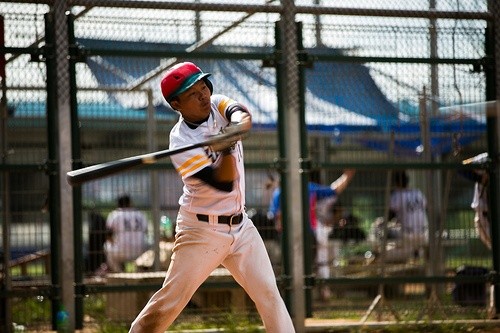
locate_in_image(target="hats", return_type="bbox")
[173,72,212,97]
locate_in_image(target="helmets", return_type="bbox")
[161,62,213,110]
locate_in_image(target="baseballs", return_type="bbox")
[66,140,213,187]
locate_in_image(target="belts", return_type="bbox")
[196,213,243,227]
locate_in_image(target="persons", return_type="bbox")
[126,62,297,333]
[388,167,431,268]
[103,195,156,274]
[267,164,357,320]
